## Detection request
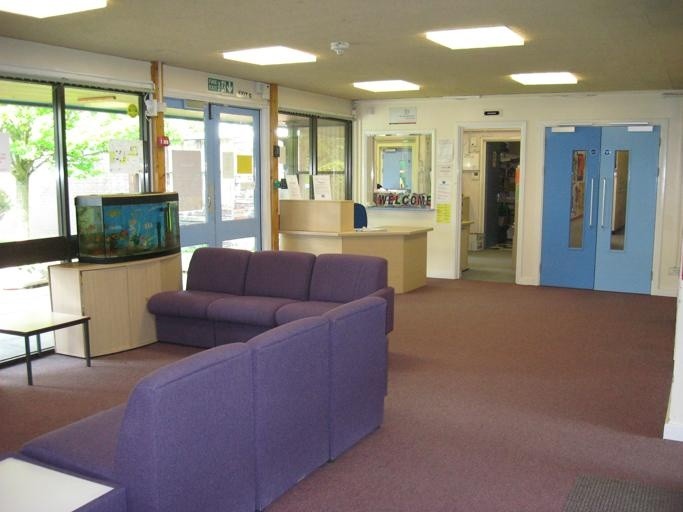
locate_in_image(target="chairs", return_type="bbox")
[354,202,367,228]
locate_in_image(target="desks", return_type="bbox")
[0,310,92,386]
[1,451,126,512]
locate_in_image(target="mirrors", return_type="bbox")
[359,129,436,212]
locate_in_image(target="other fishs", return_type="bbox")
[111,231,128,241]
[105,209,122,218]
[142,223,154,229]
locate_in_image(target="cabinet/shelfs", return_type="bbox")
[461,196,470,222]
[460,218,475,272]
[47,251,183,359]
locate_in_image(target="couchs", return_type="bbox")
[22,294,394,511]
[146,245,396,350]
